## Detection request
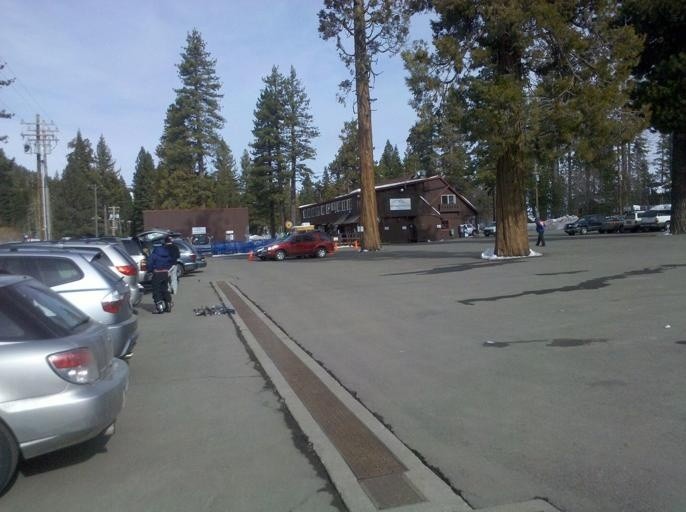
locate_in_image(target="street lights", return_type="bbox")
[87,183,105,237]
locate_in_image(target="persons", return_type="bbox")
[536,218,546,246]
[146,239,175,314]
[164,237,180,294]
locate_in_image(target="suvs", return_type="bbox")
[563,203,671,235]
[257,230,335,261]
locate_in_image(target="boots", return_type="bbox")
[152,301,170,314]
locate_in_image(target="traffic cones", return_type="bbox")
[353,240,360,248]
[248,249,256,261]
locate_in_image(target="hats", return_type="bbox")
[153,240,162,247]
[165,239,172,245]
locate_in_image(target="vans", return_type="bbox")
[484,221,496,236]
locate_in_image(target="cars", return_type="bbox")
[459,223,477,237]
